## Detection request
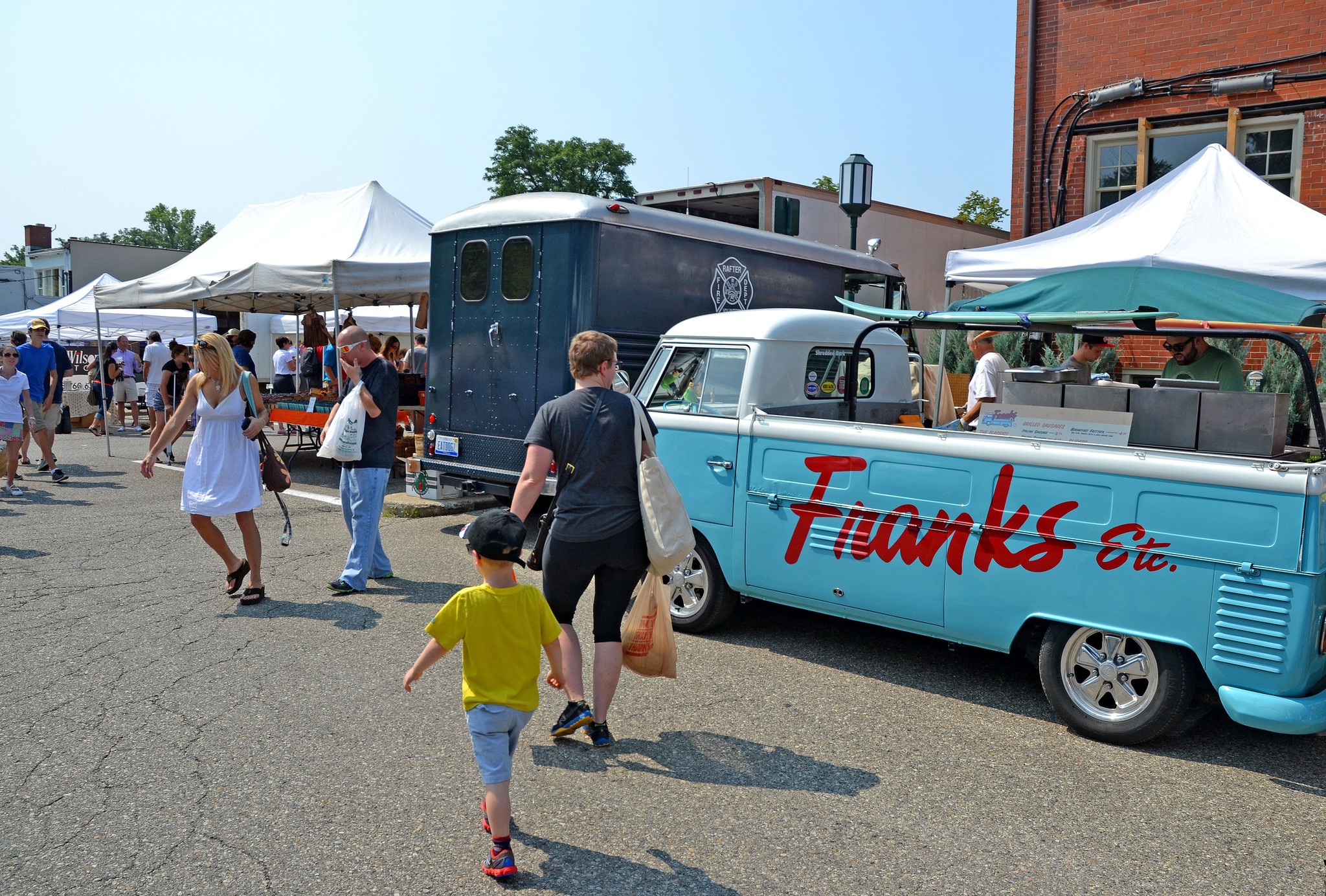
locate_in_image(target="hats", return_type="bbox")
[225,328,241,336]
[459,508,527,569]
[27,318,49,330]
[1082,334,1116,347]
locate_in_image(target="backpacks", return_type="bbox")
[300,347,322,378]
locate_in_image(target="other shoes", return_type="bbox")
[88,423,154,437]
[4,449,69,497]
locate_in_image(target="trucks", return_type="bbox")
[419,191,919,517]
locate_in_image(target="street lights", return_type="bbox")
[839,147,873,323]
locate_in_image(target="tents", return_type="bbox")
[270,301,438,344]
[925,142,1326,431]
[0,272,222,357]
[94,179,447,456]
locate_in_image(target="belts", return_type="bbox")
[123,376,134,378]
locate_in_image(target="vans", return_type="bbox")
[624,307,1326,744]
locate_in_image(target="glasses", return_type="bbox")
[285,341,290,344]
[193,338,216,350]
[1162,336,1196,352]
[337,340,367,353]
[2,352,19,358]
[607,359,623,371]
[392,346,400,350]
[177,352,191,357]
[31,328,46,332]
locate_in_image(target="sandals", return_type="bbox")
[154,448,175,464]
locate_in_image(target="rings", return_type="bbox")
[135,356,136,357]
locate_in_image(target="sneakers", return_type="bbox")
[481,796,518,880]
[325,579,355,593]
[550,698,611,747]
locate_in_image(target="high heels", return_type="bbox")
[226,558,265,606]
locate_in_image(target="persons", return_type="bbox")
[503,329,664,749]
[319,326,398,594]
[138,333,272,605]
[188,313,430,448]
[958,329,1014,434]
[1150,333,1248,394]
[7,330,33,465]
[86,341,125,436]
[33,318,73,471]
[109,335,143,432]
[0,345,37,496]
[2,319,70,483]
[402,507,565,883]
[146,340,194,464]
[142,331,174,435]
[1056,334,1117,387]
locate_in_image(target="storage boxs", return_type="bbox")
[397,455,463,500]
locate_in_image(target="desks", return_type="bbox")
[268,409,407,470]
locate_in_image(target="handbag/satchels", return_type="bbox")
[317,381,370,461]
[87,383,100,405]
[621,572,679,680]
[637,457,696,577]
[526,507,557,571]
[260,444,291,493]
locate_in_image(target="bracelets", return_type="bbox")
[259,417,266,428]
[118,366,123,368]
[138,360,142,363]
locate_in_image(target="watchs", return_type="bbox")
[29,417,36,420]
[118,367,123,371]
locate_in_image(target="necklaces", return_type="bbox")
[208,375,223,391]
